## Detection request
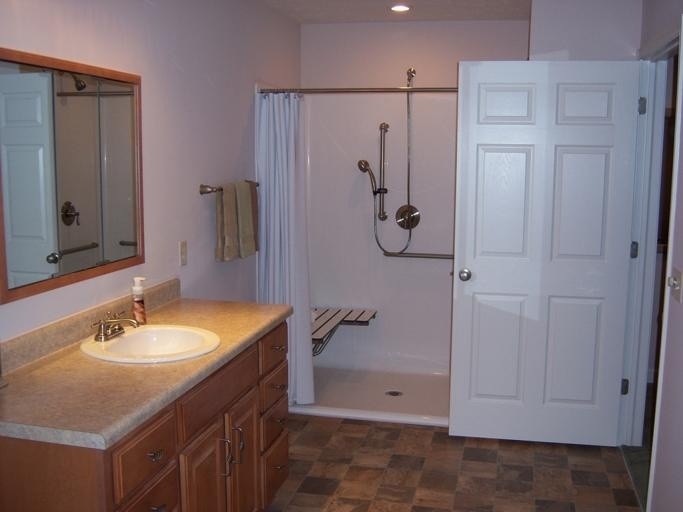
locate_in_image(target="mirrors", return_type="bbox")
[0,48,145,306]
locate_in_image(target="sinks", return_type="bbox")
[79,323,221,364]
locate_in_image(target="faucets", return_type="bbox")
[91,317,140,342]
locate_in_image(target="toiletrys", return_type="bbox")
[131,276,147,325]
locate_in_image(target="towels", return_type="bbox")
[244,179,260,251]
[234,181,255,259]
[216,183,238,261]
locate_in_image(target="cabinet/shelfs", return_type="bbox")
[175,344,259,512]
[0,408,181,512]
[257,322,289,512]
